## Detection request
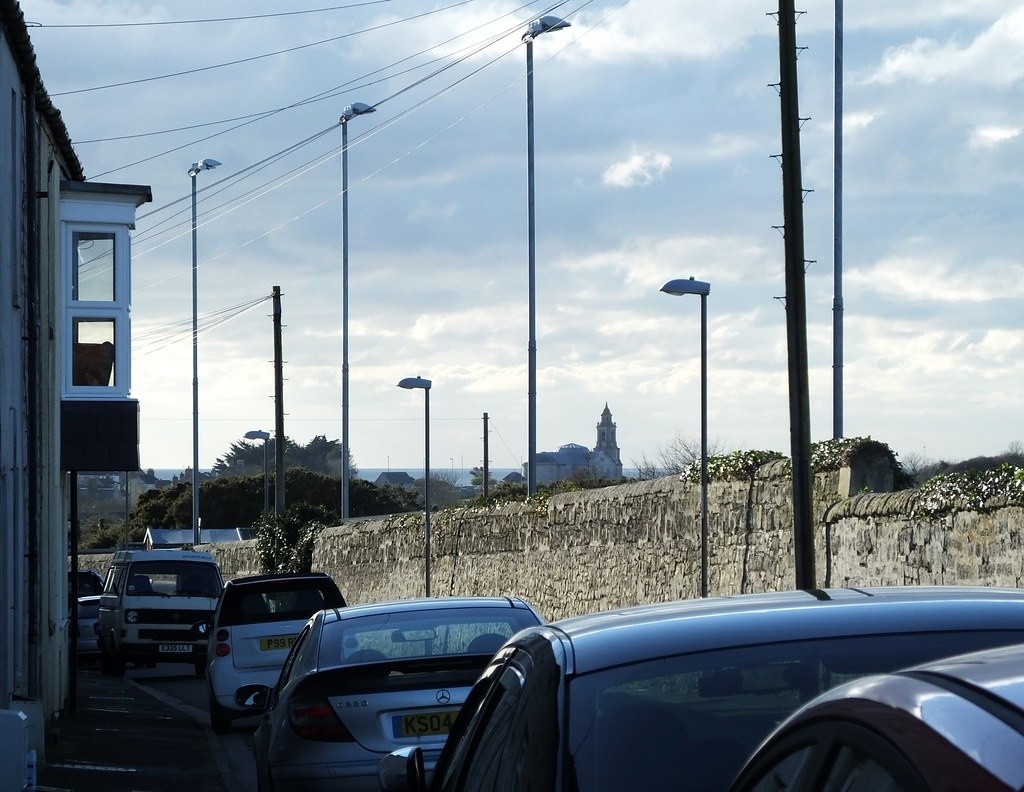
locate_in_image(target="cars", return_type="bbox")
[192,570,361,735]
[382,582,1024,791]
[232,594,545,792]
[729,644,1024,792]
[70,571,108,663]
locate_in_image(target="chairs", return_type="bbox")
[134,575,154,594]
[178,573,205,594]
[467,633,509,653]
[343,649,389,665]
[240,594,270,618]
[293,590,326,611]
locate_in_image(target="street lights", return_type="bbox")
[521,14,571,497]
[244,431,271,516]
[342,105,374,512]
[657,277,714,595]
[398,377,431,596]
[188,157,219,547]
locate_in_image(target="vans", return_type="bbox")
[97,548,226,679]
